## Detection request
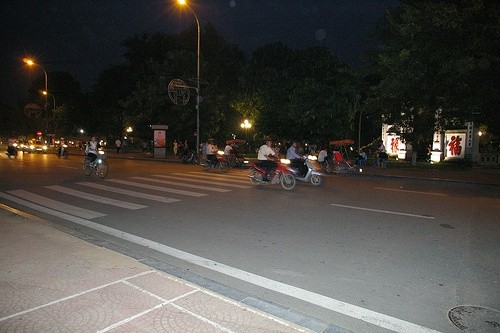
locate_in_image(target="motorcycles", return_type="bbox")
[248,158,296,191]
[284,155,323,186]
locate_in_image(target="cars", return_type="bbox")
[13,139,48,155]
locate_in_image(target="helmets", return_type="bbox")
[208,139,214,143]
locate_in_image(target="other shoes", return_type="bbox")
[303,173,306,177]
[89,165,92,166]
[262,177,270,182]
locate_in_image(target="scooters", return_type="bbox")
[81,149,109,178]
[177,145,245,169]
[5,142,18,159]
[55,144,69,159]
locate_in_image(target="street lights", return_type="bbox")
[26,60,48,140]
[42,91,56,111]
[177,0,200,156]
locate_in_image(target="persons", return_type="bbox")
[205,140,218,168]
[172,140,178,160]
[184,139,189,157]
[286,142,306,178]
[143,140,148,153]
[59,138,65,156]
[78,140,83,150]
[354,150,367,166]
[257,138,279,182]
[333,149,353,169]
[318,147,331,175]
[115,138,121,153]
[7,135,17,154]
[177,140,182,156]
[122,139,128,154]
[87,135,100,175]
[379,139,387,168]
[310,147,315,154]
[224,144,233,158]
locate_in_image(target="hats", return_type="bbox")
[266,137,273,142]
